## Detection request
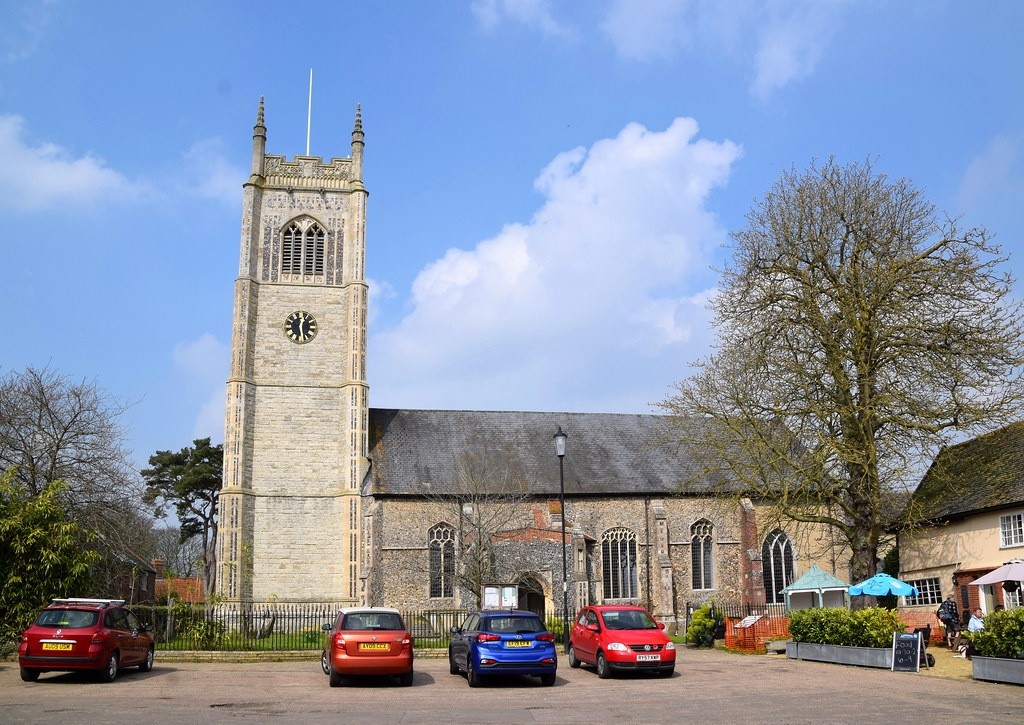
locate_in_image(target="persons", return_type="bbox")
[967,605,1005,656]
[944,594,962,650]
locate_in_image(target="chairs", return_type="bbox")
[348,618,361,629]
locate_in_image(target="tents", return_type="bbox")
[776,565,853,615]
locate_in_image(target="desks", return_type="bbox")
[953,628,968,653]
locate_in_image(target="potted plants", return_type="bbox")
[787,606,906,668]
[969,606,1024,685]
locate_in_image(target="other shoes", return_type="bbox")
[947,646,952,650]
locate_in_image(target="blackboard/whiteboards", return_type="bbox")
[892,632,928,671]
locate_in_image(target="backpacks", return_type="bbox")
[937,600,952,624]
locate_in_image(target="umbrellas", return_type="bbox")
[968,558,1024,607]
[849,574,919,612]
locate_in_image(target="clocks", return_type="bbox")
[284,310,318,344]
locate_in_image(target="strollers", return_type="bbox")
[903,624,935,667]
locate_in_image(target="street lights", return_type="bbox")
[552,425,569,654]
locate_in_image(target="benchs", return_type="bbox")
[947,636,967,652]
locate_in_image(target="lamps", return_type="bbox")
[951,575,958,586]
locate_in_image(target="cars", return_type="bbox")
[17,598,155,683]
[448,610,558,688]
[568,605,677,679]
[321,606,414,687]
